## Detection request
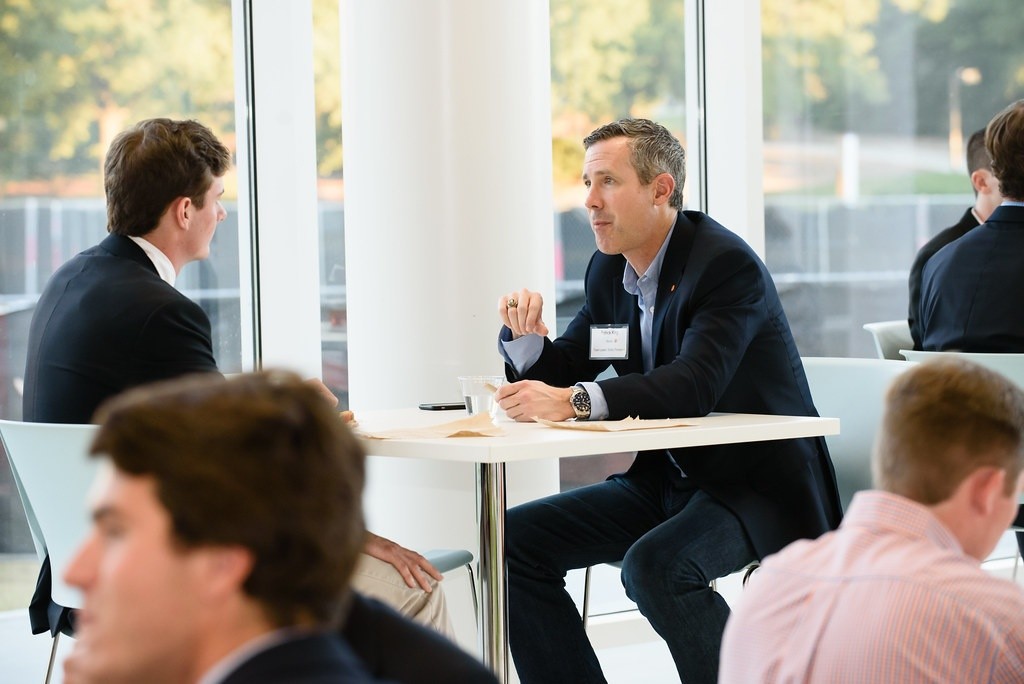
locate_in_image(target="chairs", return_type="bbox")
[582,357,920,632]
[2,420,122,684]
[897,349,1024,535]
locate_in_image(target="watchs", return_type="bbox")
[567,385,591,420]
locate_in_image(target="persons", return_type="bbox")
[19,118,460,648]
[714,354,1024,683]
[907,128,1003,355]
[494,119,846,684]
[340,440,508,684]
[61,370,370,684]
[918,98,1024,562]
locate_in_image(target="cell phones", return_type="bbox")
[419,402,467,411]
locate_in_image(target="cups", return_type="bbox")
[457,374,506,421]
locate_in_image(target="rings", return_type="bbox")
[506,298,518,308]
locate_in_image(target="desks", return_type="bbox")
[336,402,841,684]
